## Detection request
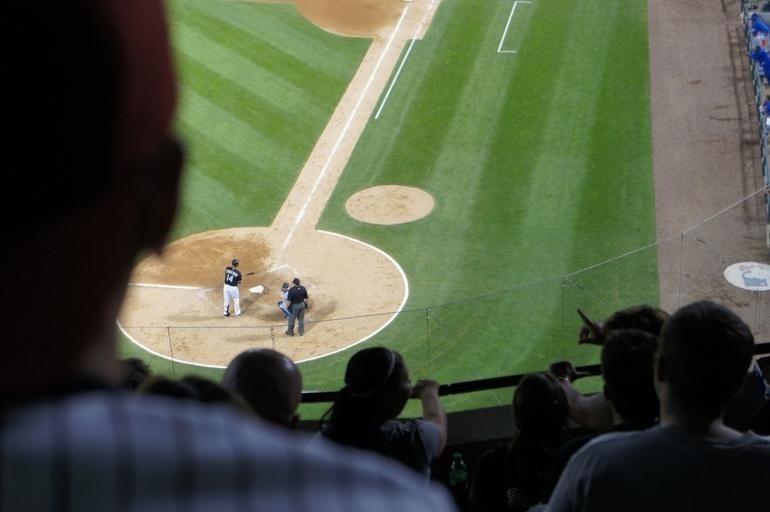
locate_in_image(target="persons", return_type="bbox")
[222,259,242,317]
[744,0,770,119]
[538,297,769,511]
[314,345,450,487]
[212,346,306,437]
[496,369,612,510]
[551,297,674,432]
[282,278,308,337]
[2,2,458,512]
[279,283,295,318]
[549,325,671,465]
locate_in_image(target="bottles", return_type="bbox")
[449,453,468,491]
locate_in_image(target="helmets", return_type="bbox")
[232,260,239,267]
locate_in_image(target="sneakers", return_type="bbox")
[224,313,230,318]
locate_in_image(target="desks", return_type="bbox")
[743,3,770,224]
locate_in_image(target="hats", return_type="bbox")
[292,278,300,283]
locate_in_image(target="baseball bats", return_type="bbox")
[241,272,255,277]
